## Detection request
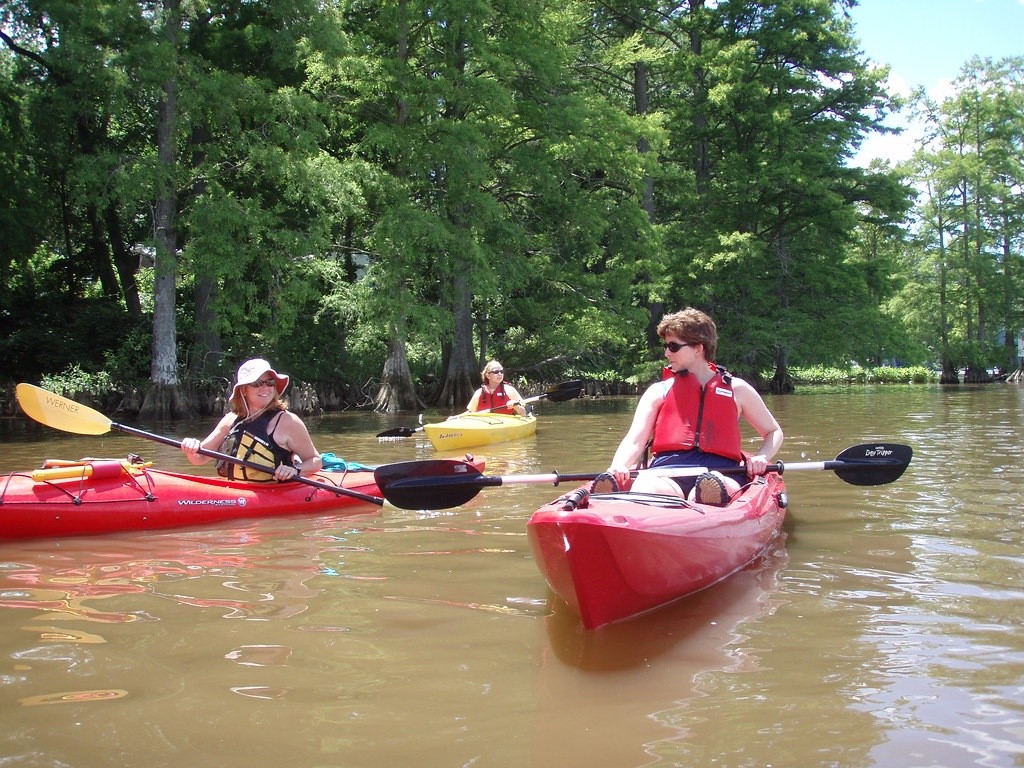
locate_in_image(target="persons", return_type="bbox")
[181,359,321,481]
[590,306,783,507]
[466,360,528,416]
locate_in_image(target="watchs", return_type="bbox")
[291,464,301,475]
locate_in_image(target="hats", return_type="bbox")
[229,359,289,415]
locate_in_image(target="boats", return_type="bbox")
[420,407,538,454]
[0,454,490,541]
[524,449,787,635]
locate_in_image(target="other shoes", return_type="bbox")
[590,473,619,494]
[695,472,728,507]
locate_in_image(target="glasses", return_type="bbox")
[488,370,504,374]
[663,342,690,352]
[248,379,275,387]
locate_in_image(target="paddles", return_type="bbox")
[375,380,583,438]
[15,383,402,513]
[373,443,914,510]
